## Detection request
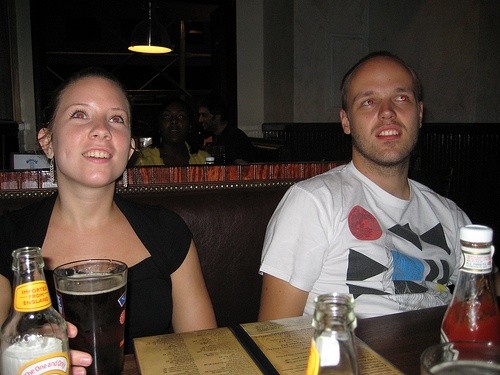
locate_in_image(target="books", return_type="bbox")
[133,313,403,374]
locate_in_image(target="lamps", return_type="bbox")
[126,0,175,55]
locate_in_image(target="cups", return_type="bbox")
[53,258,128,375]
[420,341,500,375]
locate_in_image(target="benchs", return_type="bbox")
[0,189,289,327]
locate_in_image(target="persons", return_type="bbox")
[135,98,212,165]
[0,67,218,353]
[256,51,500,322]
[198,93,255,163]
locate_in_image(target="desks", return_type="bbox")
[122,295,500,374]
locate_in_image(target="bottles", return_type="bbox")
[305,294,360,375]
[0,246,70,375]
[440,224,500,360]
[205,157,215,164]
